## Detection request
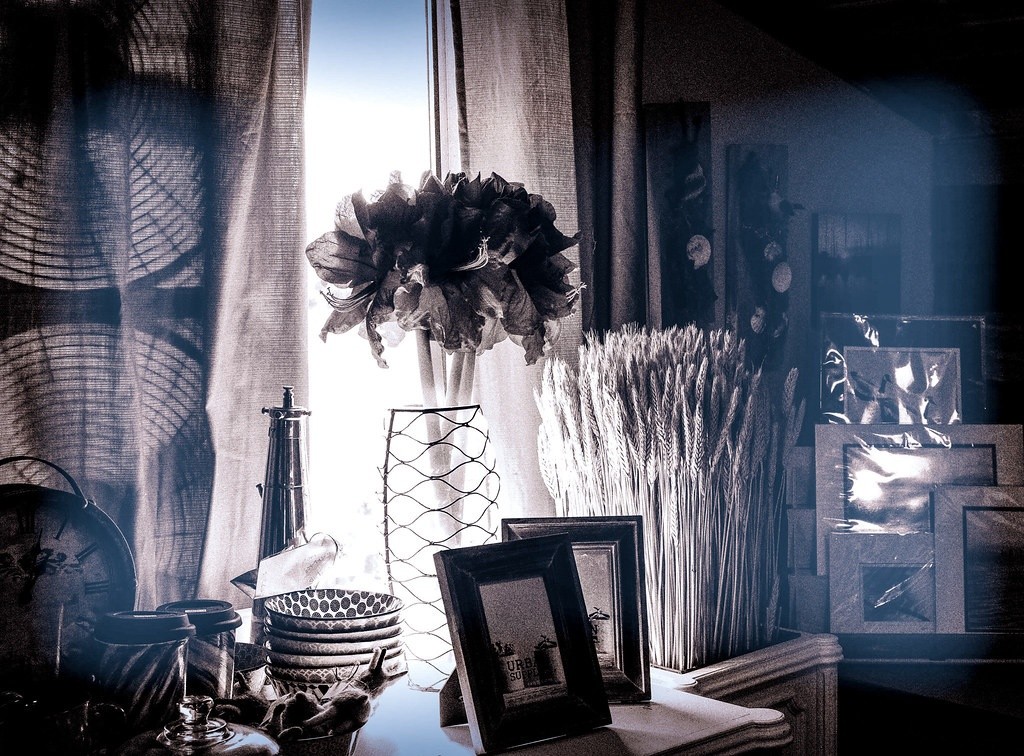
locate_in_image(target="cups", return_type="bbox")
[89,609,197,730]
[156,599,245,702]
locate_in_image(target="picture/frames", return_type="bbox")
[818,312,989,424]
[433,532,613,756]
[502,515,652,703]
[935,484,1024,634]
[815,424,1024,578]
[828,531,937,633]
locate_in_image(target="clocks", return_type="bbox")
[1,455,139,676]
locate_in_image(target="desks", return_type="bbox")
[350,628,796,756]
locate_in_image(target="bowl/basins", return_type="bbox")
[230,642,268,696]
[278,719,367,756]
[263,588,407,709]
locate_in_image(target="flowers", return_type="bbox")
[305,170,585,402]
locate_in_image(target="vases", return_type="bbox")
[376,403,500,691]
